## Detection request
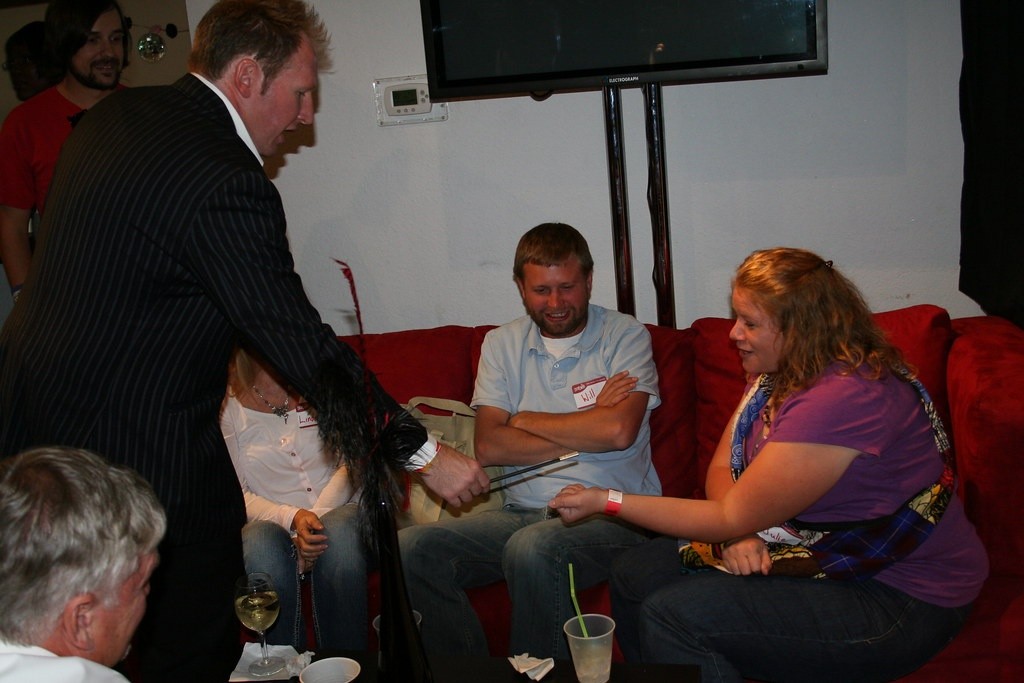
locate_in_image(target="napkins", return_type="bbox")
[507,653,554,681]
[228,642,316,682]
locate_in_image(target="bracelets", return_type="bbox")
[404,433,442,472]
[603,488,622,516]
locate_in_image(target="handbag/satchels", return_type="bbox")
[395,395,506,527]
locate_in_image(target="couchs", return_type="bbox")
[217,303,1024,683]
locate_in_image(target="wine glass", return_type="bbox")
[234,571,286,677]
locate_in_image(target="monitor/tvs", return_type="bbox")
[420,0,828,103]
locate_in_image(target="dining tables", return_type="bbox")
[224,644,702,683]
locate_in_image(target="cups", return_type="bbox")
[372,610,422,668]
[563,613,616,683]
[299,656,361,683]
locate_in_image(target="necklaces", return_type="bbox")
[252,385,289,417]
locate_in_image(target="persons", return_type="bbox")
[549,246,988,683]
[398,222,663,683]
[0,0,133,307]
[0,0,490,683]
[1,447,168,683]
[218,351,380,683]
